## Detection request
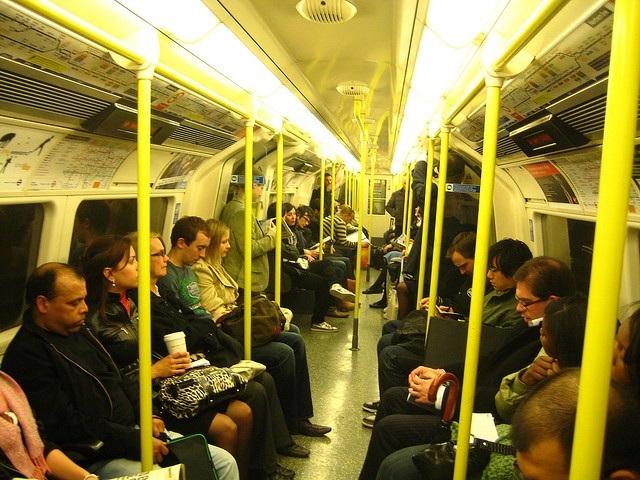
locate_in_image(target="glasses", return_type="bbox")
[487,264,502,272]
[150,251,166,257]
[302,216,311,223]
[514,295,548,307]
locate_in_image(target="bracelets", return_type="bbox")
[84,474,98,480]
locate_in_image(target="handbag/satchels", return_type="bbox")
[215,295,286,349]
[411,433,516,480]
[386,257,404,321]
[229,359,267,384]
[156,365,249,420]
[158,431,220,480]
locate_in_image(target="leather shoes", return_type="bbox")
[362,288,383,294]
[269,471,291,480]
[276,437,310,458]
[369,302,387,308]
[288,418,331,436]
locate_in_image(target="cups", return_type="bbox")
[162,332,189,359]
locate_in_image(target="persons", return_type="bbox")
[312,165,343,215]
[358,257,575,480]
[322,205,370,282]
[0,370,99,480]
[362,206,422,309]
[293,205,355,280]
[377,293,588,473]
[78,236,255,473]
[381,231,476,337]
[510,365,640,480]
[306,198,326,241]
[157,216,332,436]
[268,202,355,332]
[361,238,533,430]
[403,150,479,301]
[0,263,240,480]
[191,219,301,335]
[221,166,276,297]
[610,309,640,383]
[124,231,310,480]
[282,203,356,317]
[385,179,410,239]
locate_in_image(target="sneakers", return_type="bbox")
[329,282,356,302]
[268,463,295,477]
[335,300,355,310]
[325,308,349,318]
[362,400,380,413]
[310,321,339,332]
[361,412,378,428]
